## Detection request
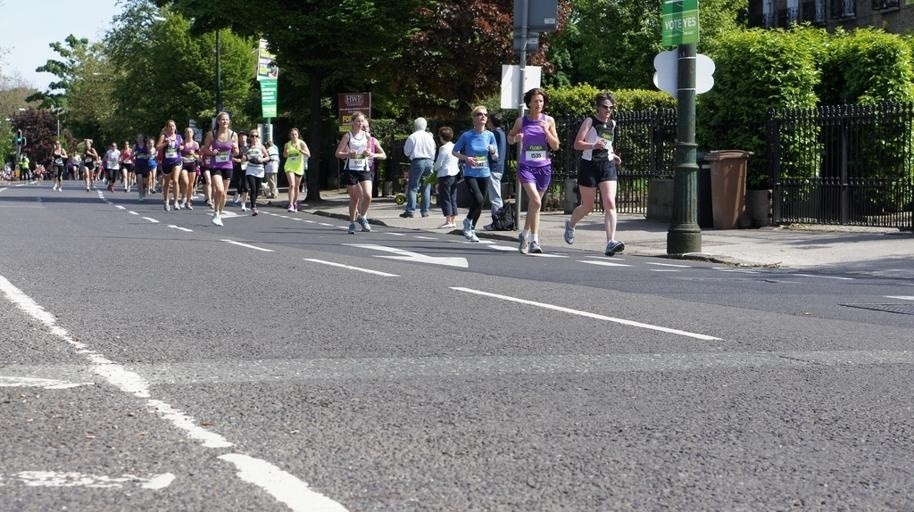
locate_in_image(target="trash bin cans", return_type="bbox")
[703,149,756,231]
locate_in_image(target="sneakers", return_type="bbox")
[400,207,542,255]
[356,215,372,232]
[52,182,298,227]
[348,222,356,234]
[605,240,624,255]
[564,219,575,244]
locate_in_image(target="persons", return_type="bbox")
[452,105,499,241]
[432,127,461,228]
[102,134,158,202]
[399,116,436,218]
[507,88,560,254]
[565,93,625,257]
[484,112,507,230]
[2,138,103,192]
[155,111,311,226]
[334,111,387,233]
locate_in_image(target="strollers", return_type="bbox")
[394,171,436,204]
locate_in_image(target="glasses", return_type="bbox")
[601,104,614,109]
[477,113,489,117]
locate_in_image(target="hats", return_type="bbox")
[238,131,250,137]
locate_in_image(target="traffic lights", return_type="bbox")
[16,131,21,145]
[21,137,26,147]
